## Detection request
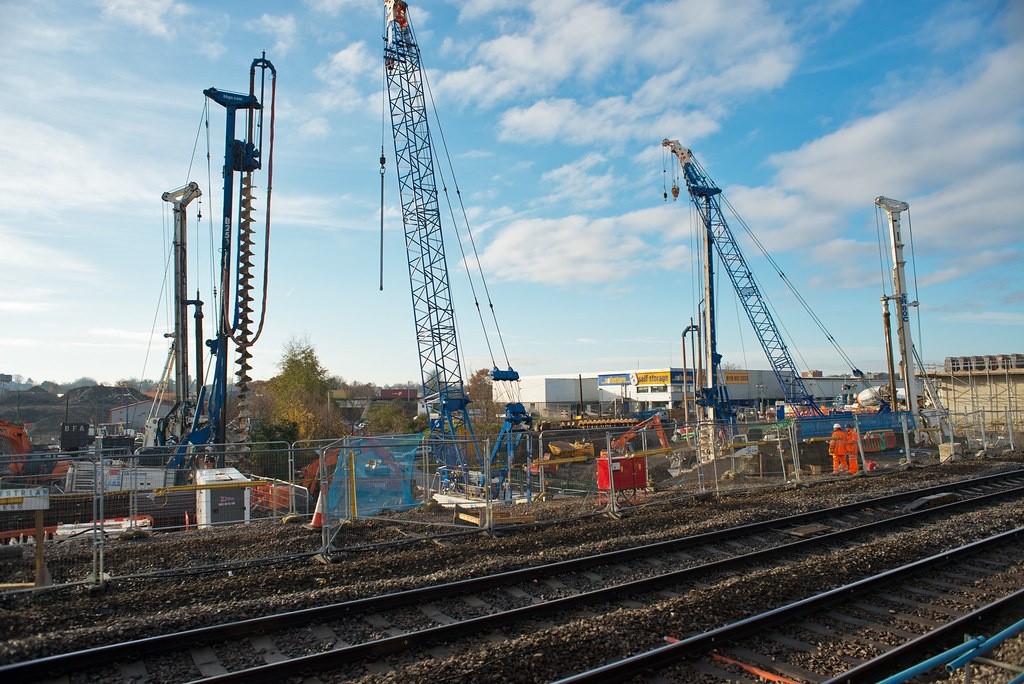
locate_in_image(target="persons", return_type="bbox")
[451,466,461,493]
[844,423,858,474]
[204,441,216,469]
[736,404,835,423]
[103,433,129,437]
[611,437,617,448]
[828,422,848,475]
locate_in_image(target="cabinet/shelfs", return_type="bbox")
[938,442,962,463]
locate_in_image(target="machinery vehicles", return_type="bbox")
[601,413,673,468]
[249,431,420,523]
[0,419,75,484]
[523,438,596,478]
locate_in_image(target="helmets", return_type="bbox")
[845,423,851,428]
[833,423,841,429]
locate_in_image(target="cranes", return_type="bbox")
[656,135,910,454]
[376,0,535,485]
[166,48,279,479]
[161,183,206,472]
[871,194,975,453]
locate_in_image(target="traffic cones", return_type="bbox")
[304,481,334,531]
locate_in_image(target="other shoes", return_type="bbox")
[839,469,846,473]
[843,470,853,475]
[829,472,839,476]
